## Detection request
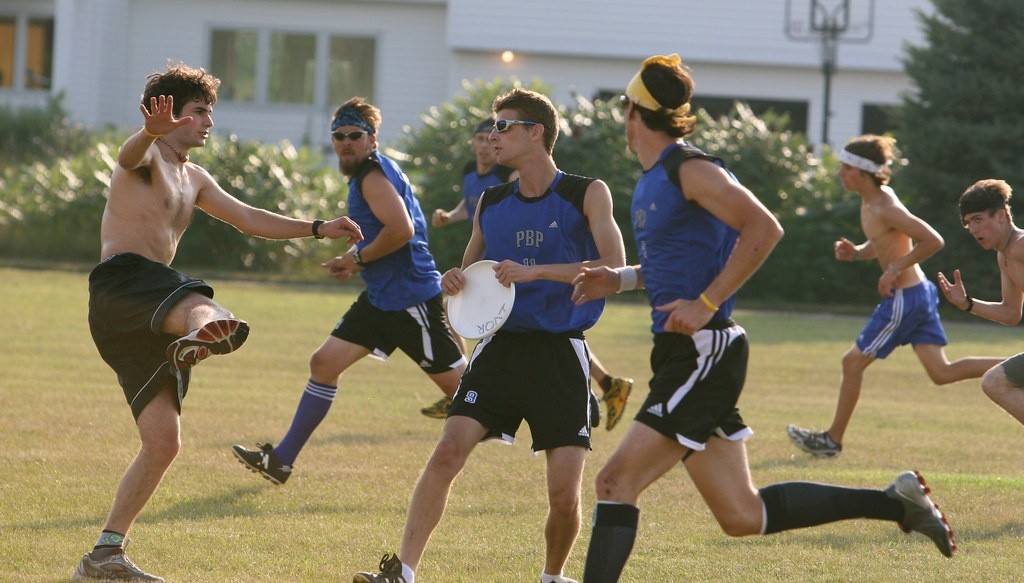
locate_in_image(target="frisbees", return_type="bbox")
[445,259,516,341]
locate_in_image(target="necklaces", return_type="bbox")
[159,135,190,163]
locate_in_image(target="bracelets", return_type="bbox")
[614,264,638,294]
[312,219,324,239]
[143,127,165,138]
[887,264,901,274]
[965,295,973,312]
[699,292,720,313]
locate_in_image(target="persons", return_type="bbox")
[786,135,1008,459]
[420,116,633,430]
[571,54,956,583]
[938,179,1024,427]
[71,64,364,582]
[232,97,468,487]
[353,89,627,583]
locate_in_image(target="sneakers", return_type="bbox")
[602,377,630,431]
[421,397,451,417]
[232,443,293,486]
[166,319,249,369]
[353,553,405,583]
[73,539,164,583]
[787,424,842,459]
[886,471,958,557]
[590,389,599,427]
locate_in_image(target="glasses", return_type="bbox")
[491,120,545,129]
[617,96,630,114]
[330,130,369,139]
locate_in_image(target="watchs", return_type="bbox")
[352,249,363,266]
